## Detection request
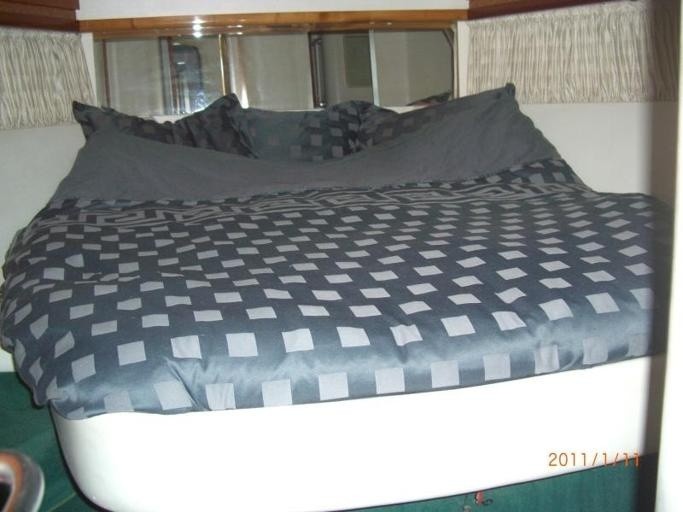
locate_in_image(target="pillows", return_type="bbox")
[70,79,520,158]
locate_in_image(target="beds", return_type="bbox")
[5,98,673,512]
